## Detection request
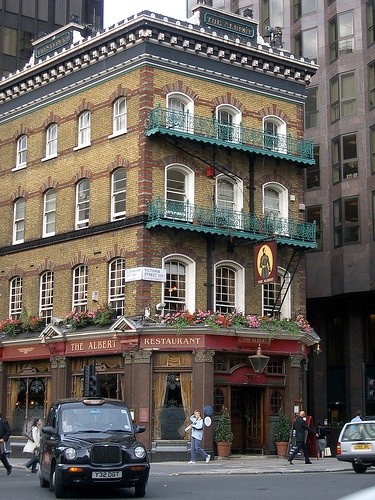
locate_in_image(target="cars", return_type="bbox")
[336,420,375,473]
[38,397,151,498]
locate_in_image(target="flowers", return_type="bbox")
[155,308,314,343]
[0,316,42,337]
[62,300,116,332]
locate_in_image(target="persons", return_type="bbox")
[22,418,42,474]
[288,410,319,465]
[351,410,363,422]
[62,410,85,433]
[187,410,211,464]
[0,416,13,476]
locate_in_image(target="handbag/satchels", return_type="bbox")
[33,447,40,457]
[0,440,12,454]
[25,429,34,443]
[185,425,192,435]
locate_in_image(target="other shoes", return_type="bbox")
[188,460,197,464]
[205,455,211,463]
[305,461,313,464]
[6,467,12,475]
[287,458,294,465]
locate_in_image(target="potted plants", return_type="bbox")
[272,405,291,456]
[215,405,235,456]
